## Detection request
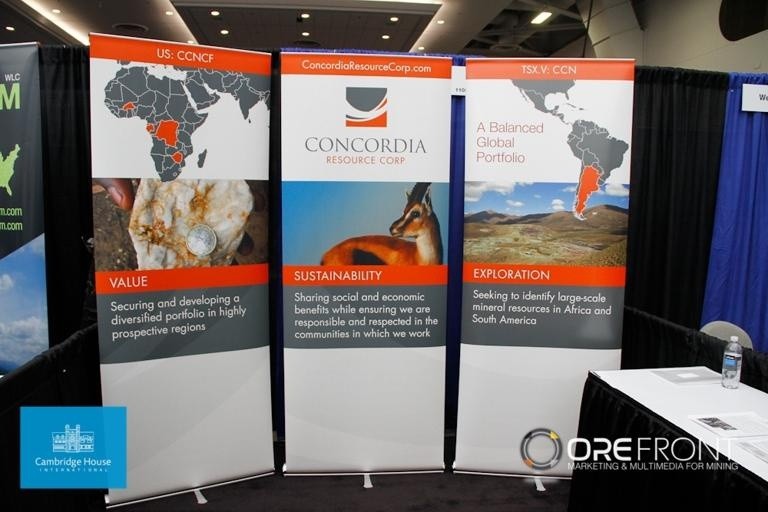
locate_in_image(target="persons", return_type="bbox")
[94,178,255,269]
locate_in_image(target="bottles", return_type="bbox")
[722,336,742,389]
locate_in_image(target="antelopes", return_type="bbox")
[321,183,443,266]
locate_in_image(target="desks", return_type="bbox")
[573,365,767,509]
